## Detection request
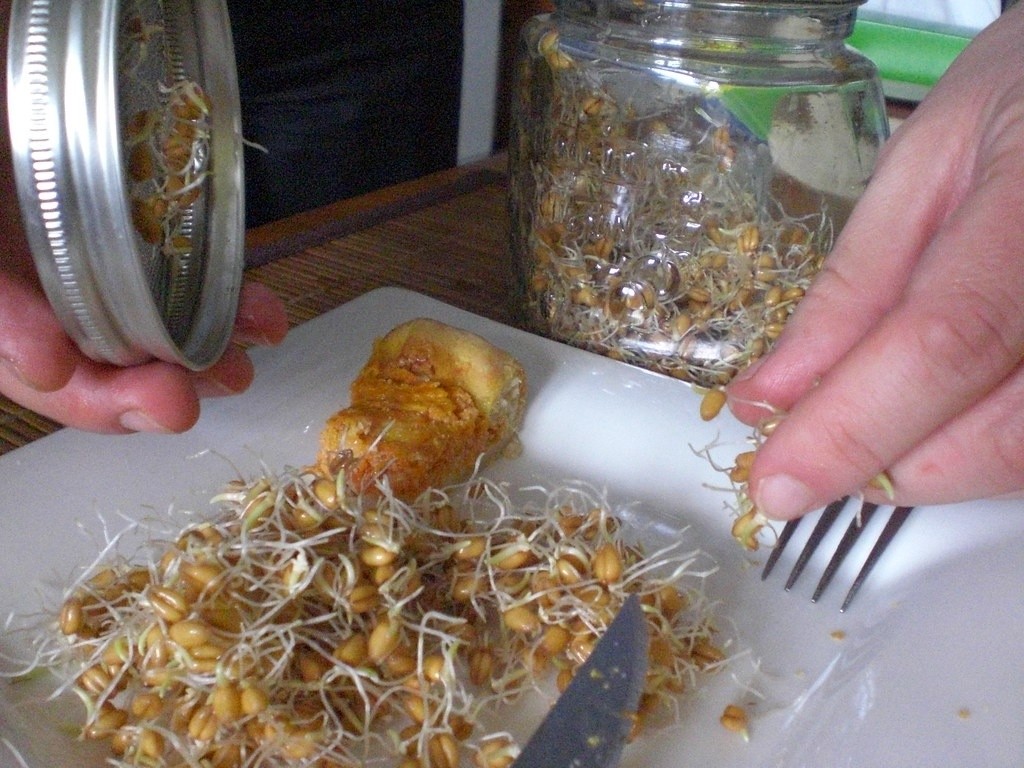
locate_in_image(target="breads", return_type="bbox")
[317,317,528,496]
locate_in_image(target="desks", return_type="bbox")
[0,162,869,768]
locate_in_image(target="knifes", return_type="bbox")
[509,591,651,768]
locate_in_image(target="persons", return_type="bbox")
[0,0,1024,521]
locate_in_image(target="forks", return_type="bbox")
[761,494,916,611]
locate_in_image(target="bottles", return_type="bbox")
[509,0,893,389]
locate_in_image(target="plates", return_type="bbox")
[1,287,1024,768]
